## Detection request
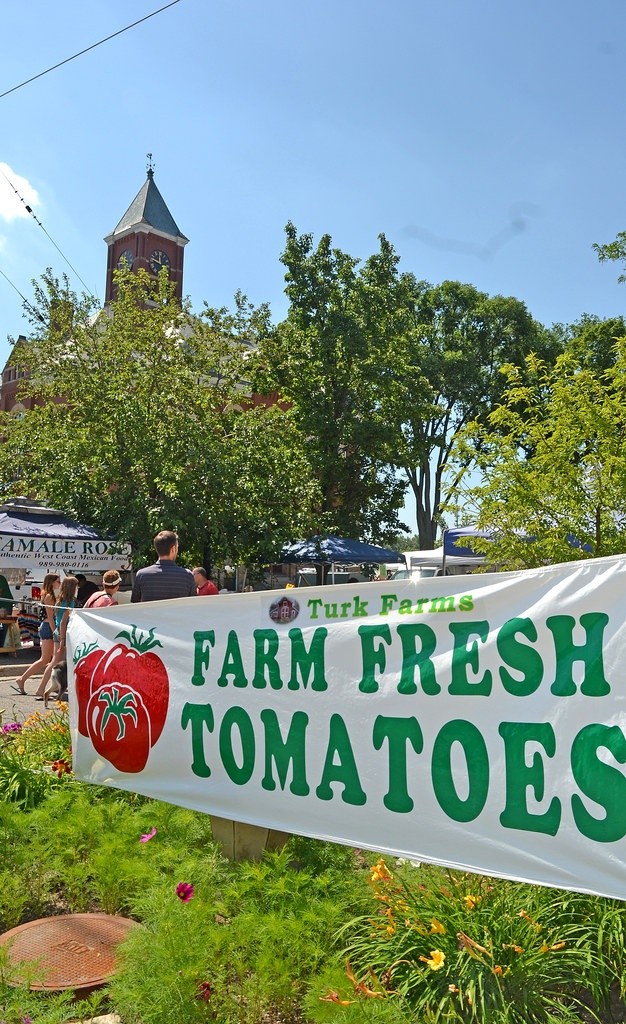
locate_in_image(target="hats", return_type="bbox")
[102,574,122,586]
[16,609,27,615]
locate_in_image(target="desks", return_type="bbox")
[0,617,19,658]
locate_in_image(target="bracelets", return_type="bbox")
[60,637,66,640]
[52,628,57,635]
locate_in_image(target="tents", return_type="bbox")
[0,496,132,654]
[237,517,594,593]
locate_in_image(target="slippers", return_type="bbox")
[34,695,54,701]
[10,684,28,695]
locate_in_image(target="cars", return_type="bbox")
[388,565,451,581]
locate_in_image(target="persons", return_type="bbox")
[82,569,122,608]
[74,574,100,606]
[387,569,392,579]
[0,573,14,648]
[16,573,61,698]
[35,576,80,700]
[192,566,219,596]
[130,530,197,603]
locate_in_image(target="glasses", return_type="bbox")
[54,580,60,583]
